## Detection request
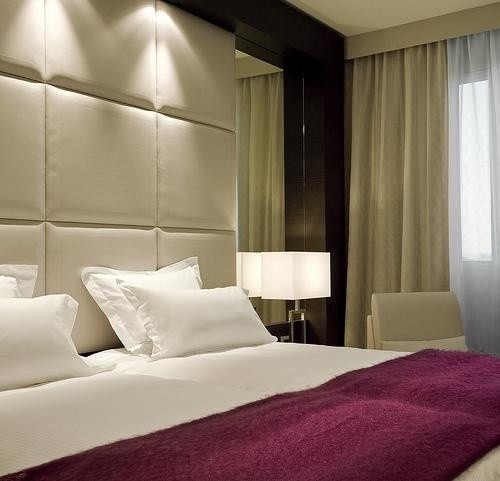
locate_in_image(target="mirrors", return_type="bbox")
[234,20,304,326]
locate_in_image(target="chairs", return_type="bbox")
[367,291,469,352]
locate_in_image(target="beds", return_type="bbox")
[0,340,500,480]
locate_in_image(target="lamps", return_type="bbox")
[259,250,331,320]
[236,251,264,298]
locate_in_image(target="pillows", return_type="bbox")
[79,255,202,356]
[113,277,278,360]
[0,263,39,301]
[0,293,116,392]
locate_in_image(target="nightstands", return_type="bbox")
[264,319,311,342]
